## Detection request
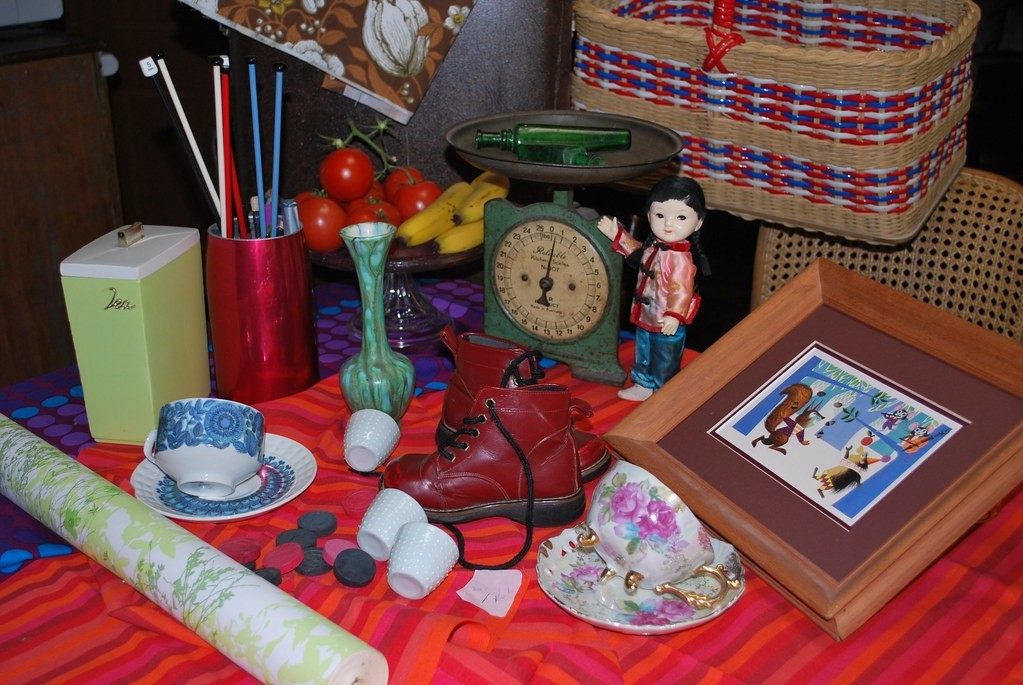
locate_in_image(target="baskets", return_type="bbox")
[568,0,983,248]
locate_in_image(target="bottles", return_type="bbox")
[475,123,632,163]
[337,221,415,429]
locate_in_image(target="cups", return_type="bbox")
[206,216,315,407]
[143,398,264,496]
[343,409,400,472]
[386,521,460,599]
[577,460,740,609]
[355,488,428,561]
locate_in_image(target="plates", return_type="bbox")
[535,521,745,635]
[130,433,318,521]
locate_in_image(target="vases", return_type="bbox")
[337,219,414,419]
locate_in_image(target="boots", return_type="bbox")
[435,323,612,483]
[378,382,586,571]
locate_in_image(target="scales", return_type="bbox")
[441,110,683,385]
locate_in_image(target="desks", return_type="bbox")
[0,271,1023,685]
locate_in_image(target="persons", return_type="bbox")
[597,175,712,402]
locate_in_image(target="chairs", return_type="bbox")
[749,170,1023,343]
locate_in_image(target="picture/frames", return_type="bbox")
[604,259,1023,647]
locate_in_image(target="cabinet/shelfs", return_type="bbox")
[0,52,122,383]
